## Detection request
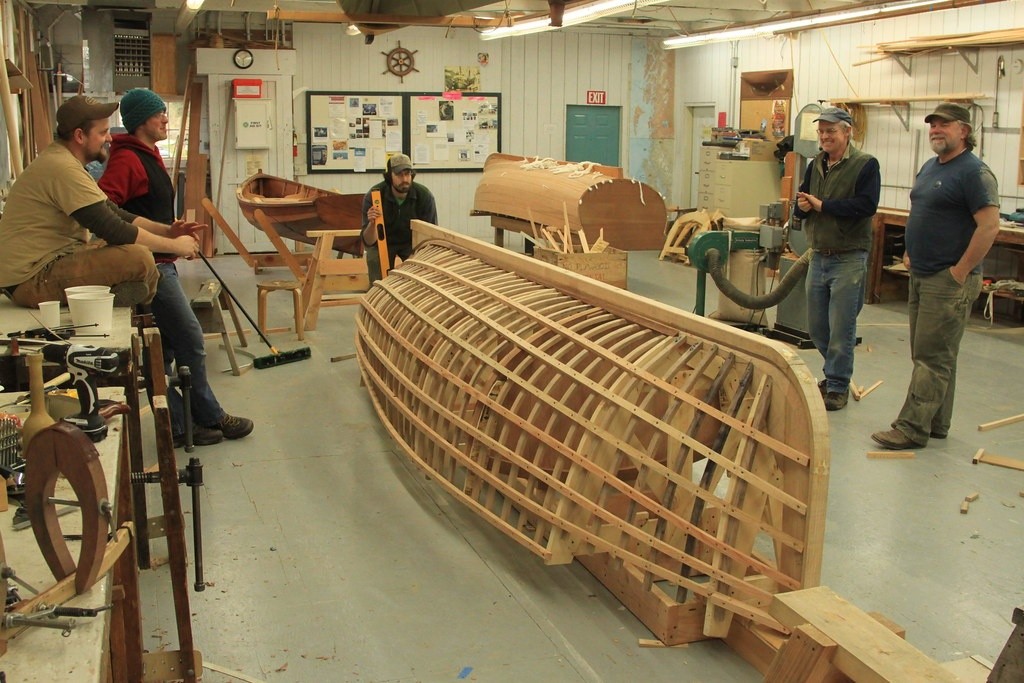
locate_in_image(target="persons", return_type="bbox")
[871,104,1000,451]
[0,96,209,308]
[358,152,438,290]
[478,53,489,63]
[97,88,254,446]
[794,106,881,412]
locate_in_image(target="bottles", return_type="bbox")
[21,352,57,459]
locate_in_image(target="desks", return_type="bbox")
[863,206,1024,305]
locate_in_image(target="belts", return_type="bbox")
[2,285,18,298]
[813,248,841,256]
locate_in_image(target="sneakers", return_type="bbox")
[173,422,222,448]
[203,412,254,440]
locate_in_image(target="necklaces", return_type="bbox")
[960,147,967,153]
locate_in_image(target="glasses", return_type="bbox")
[817,127,847,134]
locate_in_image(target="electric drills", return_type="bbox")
[19,344,119,442]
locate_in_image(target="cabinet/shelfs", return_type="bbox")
[696,141,781,222]
[0,292,145,683]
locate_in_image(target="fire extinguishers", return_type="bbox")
[292,130,297,157]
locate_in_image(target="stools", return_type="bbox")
[256,279,305,342]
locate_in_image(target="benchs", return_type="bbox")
[186,279,247,376]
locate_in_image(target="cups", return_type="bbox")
[38,285,116,334]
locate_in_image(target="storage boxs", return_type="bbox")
[533,243,629,291]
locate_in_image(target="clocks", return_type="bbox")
[233,49,254,69]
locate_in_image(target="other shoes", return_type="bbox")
[824,388,849,411]
[871,429,917,449]
[817,379,827,396]
[930,430,947,439]
[109,280,149,308]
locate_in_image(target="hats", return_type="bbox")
[925,102,970,124]
[812,107,851,125]
[119,87,166,133]
[55,95,119,132]
[388,154,414,175]
